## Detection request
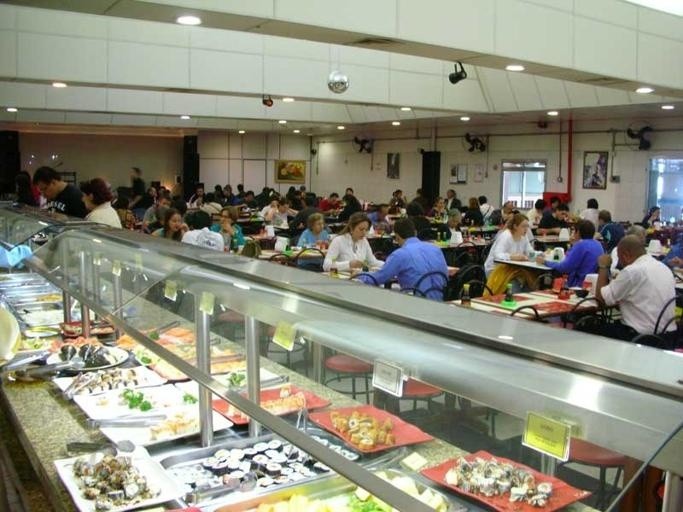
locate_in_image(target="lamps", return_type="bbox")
[449,61,466,86]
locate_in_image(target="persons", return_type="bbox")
[535,216,605,290]
[573,232,679,352]
[114,160,682,275]
[353,216,450,302]
[483,212,545,294]
[12,161,123,230]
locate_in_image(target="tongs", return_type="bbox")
[187,353,246,367]
[288,407,311,465]
[2,348,86,381]
[240,375,289,389]
[165,472,259,509]
[85,415,168,430]
[61,371,101,401]
[67,441,136,458]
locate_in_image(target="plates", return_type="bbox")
[59,321,116,338]
[46,344,129,371]
[420,449,593,511]
[309,404,435,453]
[118,328,196,352]
[135,342,222,367]
[175,367,283,402]
[97,402,234,445]
[74,384,186,420]
[149,351,247,381]
[52,364,170,397]
[54,444,186,512]
[210,383,331,426]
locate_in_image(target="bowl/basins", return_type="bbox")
[574,289,588,297]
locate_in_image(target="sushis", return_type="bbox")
[183,434,361,491]
[77,369,138,394]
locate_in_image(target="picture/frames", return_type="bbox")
[274,159,305,184]
[581,151,608,191]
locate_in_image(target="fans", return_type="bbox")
[624,120,656,153]
[461,130,486,156]
[351,133,372,154]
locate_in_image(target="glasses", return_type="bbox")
[221,215,230,219]
[521,224,530,228]
[40,184,48,193]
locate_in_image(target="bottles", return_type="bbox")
[461,284,470,306]
[435,232,440,245]
[441,232,448,245]
[329,257,337,277]
[361,255,370,271]
[504,282,514,300]
[284,239,291,255]
[554,249,560,260]
